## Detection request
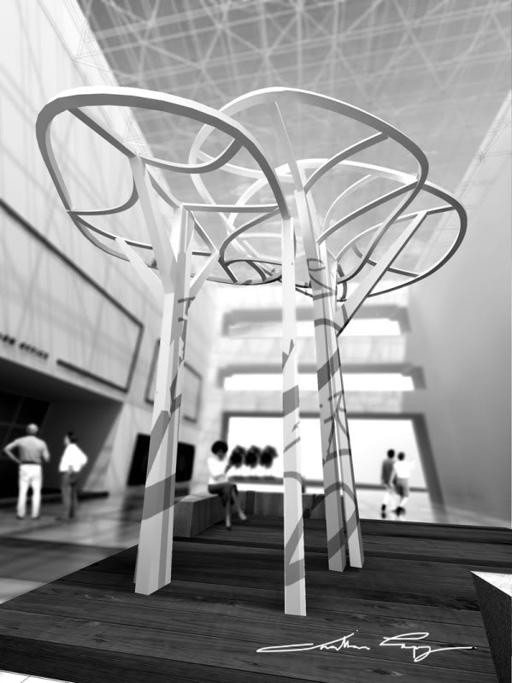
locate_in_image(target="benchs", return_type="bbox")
[170,482,347,544]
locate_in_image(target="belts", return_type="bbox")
[20,459,37,466]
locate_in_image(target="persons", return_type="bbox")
[54,430,88,521]
[207,440,249,530]
[3,422,51,520]
[388,451,410,513]
[380,448,397,512]
